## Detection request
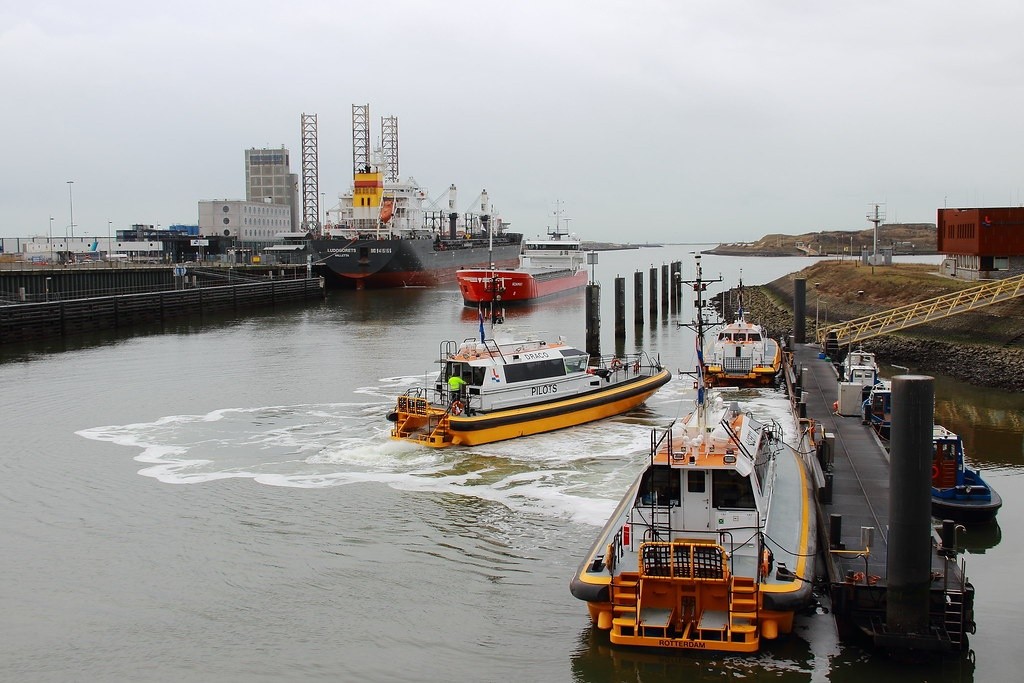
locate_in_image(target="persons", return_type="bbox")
[326,232,432,241]
[64,261,68,267]
[438,241,445,251]
[448,371,466,402]
[459,266,465,270]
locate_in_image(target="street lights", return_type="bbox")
[66,224,78,269]
[49,217,54,265]
[108,221,113,262]
[157,224,161,264]
[66,181,74,241]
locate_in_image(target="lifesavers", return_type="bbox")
[610,359,622,370]
[932,466,940,479]
[56,292,60,300]
[761,548,769,578]
[452,401,463,415]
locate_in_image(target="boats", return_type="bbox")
[569,250,831,655]
[836,342,910,440]
[455,205,589,313]
[927,424,1003,527]
[386,297,674,450]
[306,136,525,293]
[693,266,782,390]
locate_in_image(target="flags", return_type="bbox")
[479,306,485,343]
